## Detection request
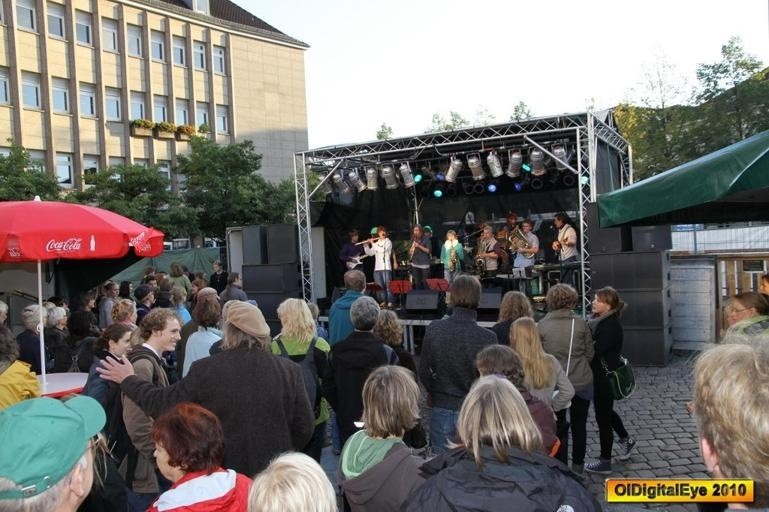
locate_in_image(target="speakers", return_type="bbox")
[330,286,373,307]
[587,203,674,366]
[241,223,301,339]
[400,290,446,320]
[477,286,503,321]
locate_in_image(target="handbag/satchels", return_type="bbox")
[552,391,572,411]
[607,357,636,400]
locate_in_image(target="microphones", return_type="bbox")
[549,225,556,232]
[382,234,386,236]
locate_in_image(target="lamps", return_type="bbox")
[444,140,569,184]
[331,162,416,194]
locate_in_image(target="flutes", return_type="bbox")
[355,236,385,246]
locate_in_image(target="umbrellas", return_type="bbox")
[0,192,166,382]
[592,126,769,234]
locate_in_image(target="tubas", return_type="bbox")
[509,225,535,258]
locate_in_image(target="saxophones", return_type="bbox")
[475,236,485,267]
[449,239,456,272]
[407,234,418,261]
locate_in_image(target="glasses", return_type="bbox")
[87,433,107,455]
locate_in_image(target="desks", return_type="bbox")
[36,371,89,398]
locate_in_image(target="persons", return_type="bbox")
[0,254,654,508]
[683,272,769,417]
[308,210,588,294]
[687,334,769,511]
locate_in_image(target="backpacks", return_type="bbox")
[106,355,160,468]
[276,336,320,419]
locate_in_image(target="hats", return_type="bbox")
[134,285,156,300]
[423,226,432,233]
[198,288,220,300]
[222,300,270,338]
[369,227,378,234]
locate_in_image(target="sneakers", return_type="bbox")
[584,459,612,475]
[380,302,386,306]
[619,435,638,460]
[388,302,393,307]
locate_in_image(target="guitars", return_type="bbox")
[346,255,368,269]
[554,236,569,258]
